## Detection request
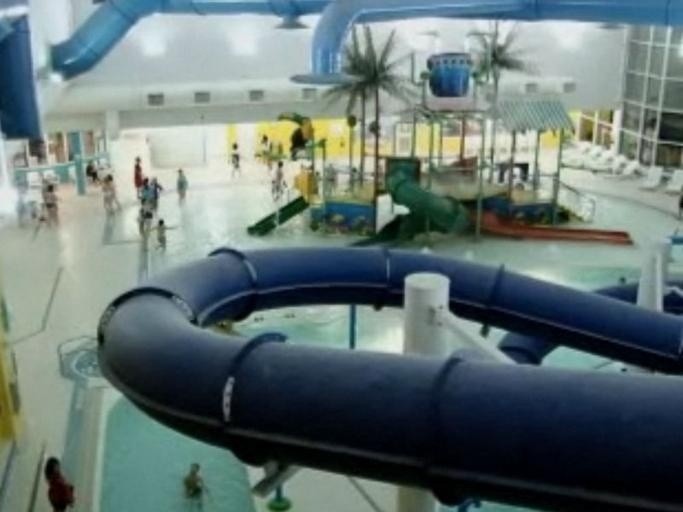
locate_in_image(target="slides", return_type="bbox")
[247,196,309,236]
[349,158,469,245]
[470,209,630,243]
[96,246,681,512]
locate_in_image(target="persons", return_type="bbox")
[229,142,241,177]
[101,174,121,215]
[176,170,187,197]
[86,159,100,181]
[182,463,208,510]
[136,178,177,252]
[134,157,143,198]
[45,456,73,512]
[270,162,287,208]
[44,184,58,224]
